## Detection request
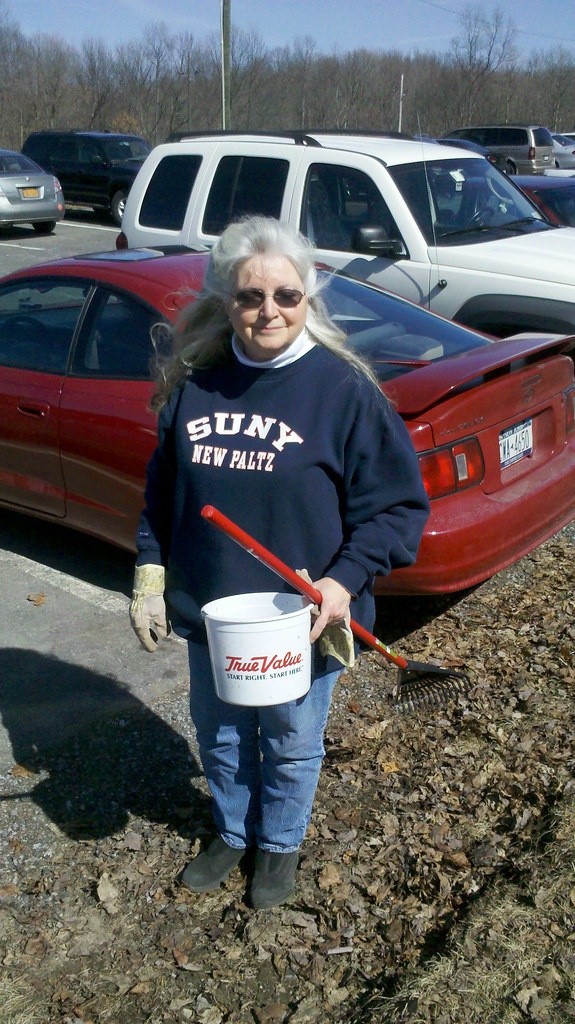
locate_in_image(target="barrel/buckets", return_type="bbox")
[200,592,314,706]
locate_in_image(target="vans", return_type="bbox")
[442,124,556,175]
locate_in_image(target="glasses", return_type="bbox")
[228,288,306,309]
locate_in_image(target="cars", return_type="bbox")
[433,138,498,169]
[0,241,575,607]
[461,175,575,229]
[0,147,67,240]
[552,132,575,171]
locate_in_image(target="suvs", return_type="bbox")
[21,128,153,227]
[114,128,574,345]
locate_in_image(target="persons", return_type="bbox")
[130,216,429,910]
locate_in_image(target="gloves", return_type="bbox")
[295,569,355,667]
[128,562,173,653]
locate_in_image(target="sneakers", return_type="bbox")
[249,847,299,911]
[180,833,251,892]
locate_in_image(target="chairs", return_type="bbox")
[308,180,352,251]
[92,299,150,371]
